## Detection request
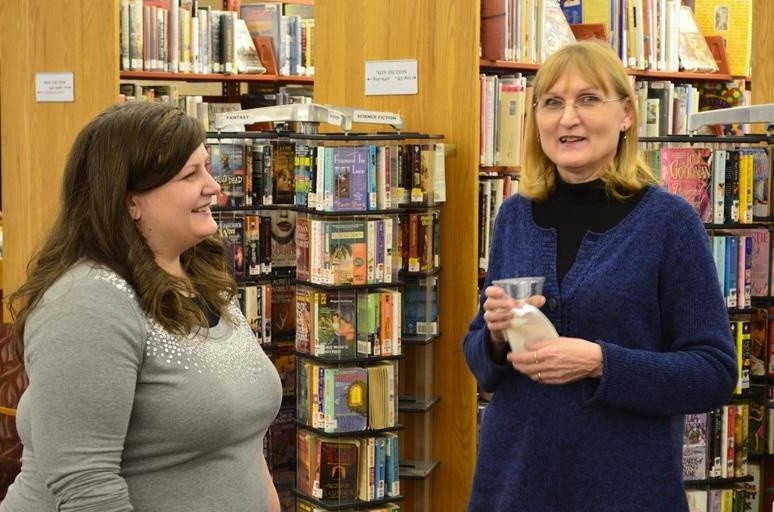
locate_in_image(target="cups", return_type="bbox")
[491,276,548,341]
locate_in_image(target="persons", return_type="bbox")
[461,40,739,512]
[2,98,287,512]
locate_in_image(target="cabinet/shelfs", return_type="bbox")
[475,0,774,512]
[117,66,314,512]
[285,131,448,511]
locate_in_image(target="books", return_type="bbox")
[480,73,772,272]
[682,227,774,511]
[261,354,401,511]
[119,0,315,76]
[121,83,446,211]
[479,0,752,78]
[211,214,441,358]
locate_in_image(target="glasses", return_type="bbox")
[533,94,625,116]
